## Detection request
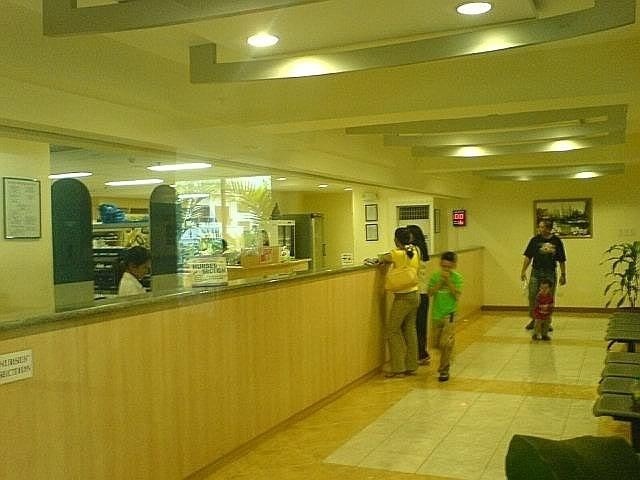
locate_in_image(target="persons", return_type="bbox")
[406,224,432,365]
[426,250,463,382]
[117,244,155,298]
[380,227,421,379]
[520,216,567,332]
[530,278,555,342]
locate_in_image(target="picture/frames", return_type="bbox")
[365,204,378,221]
[366,224,378,241]
[3,176,42,239]
[434,208,441,235]
[533,198,593,239]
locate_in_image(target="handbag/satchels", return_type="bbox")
[385,265,419,292]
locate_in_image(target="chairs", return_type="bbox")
[593,313,639,451]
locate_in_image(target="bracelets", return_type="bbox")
[561,272,565,277]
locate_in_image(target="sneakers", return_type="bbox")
[438,370,450,381]
[383,357,432,379]
[525,320,554,342]
